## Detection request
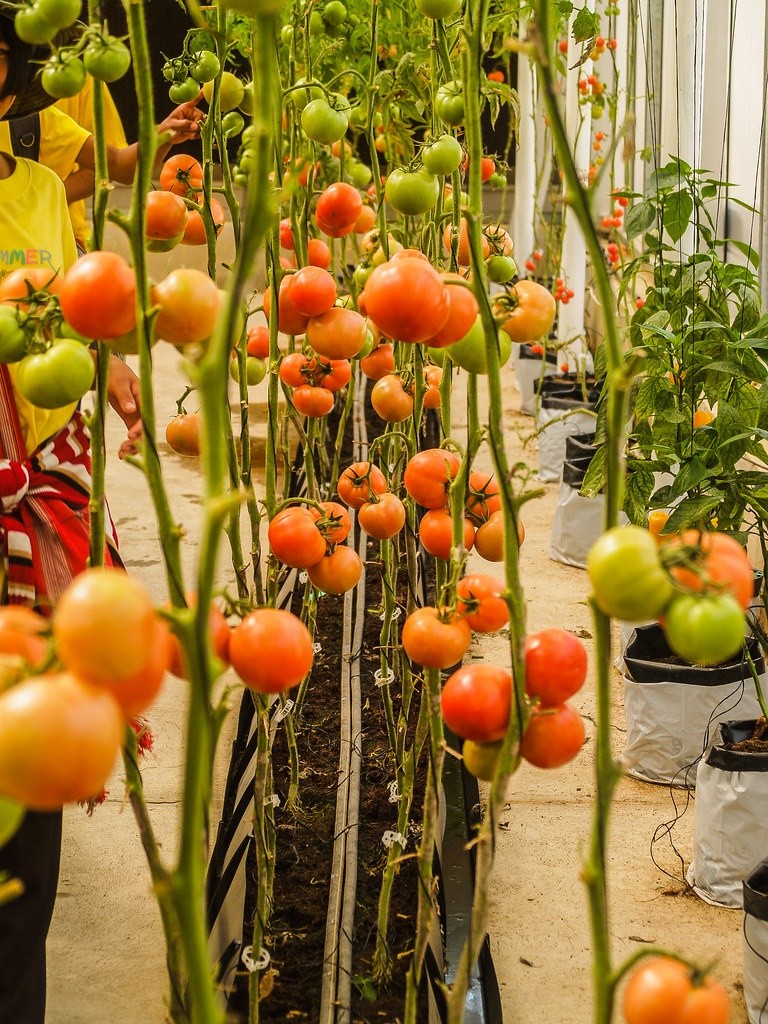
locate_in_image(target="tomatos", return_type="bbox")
[0,0,751,1024]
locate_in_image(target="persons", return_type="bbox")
[2,0,211,1024]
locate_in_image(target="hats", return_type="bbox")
[2,2,88,121]
